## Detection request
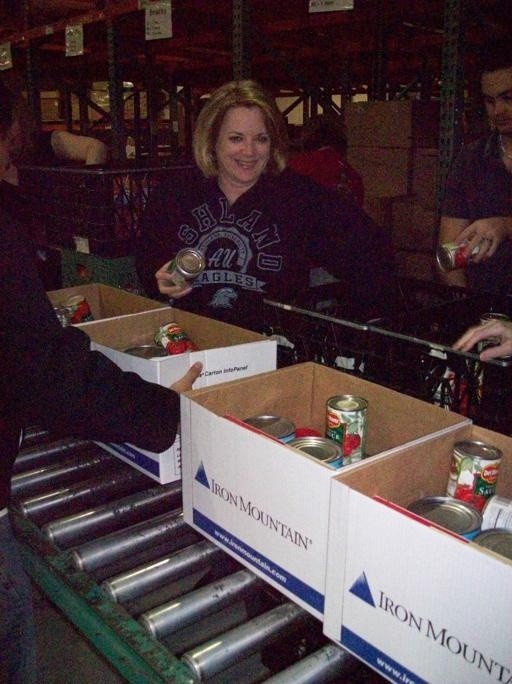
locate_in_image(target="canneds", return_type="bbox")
[435,237,481,274]
[325,394,368,466]
[153,321,200,355]
[406,439,511,561]
[122,344,168,359]
[241,414,296,444]
[477,312,510,354]
[164,247,206,289]
[59,293,95,324]
[286,436,344,470]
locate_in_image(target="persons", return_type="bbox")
[269,107,363,320]
[0,87,204,683]
[3,125,108,187]
[133,79,394,357]
[450,316,511,365]
[435,50,512,338]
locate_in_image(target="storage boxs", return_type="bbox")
[11,280,511,672]
[338,97,442,278]
